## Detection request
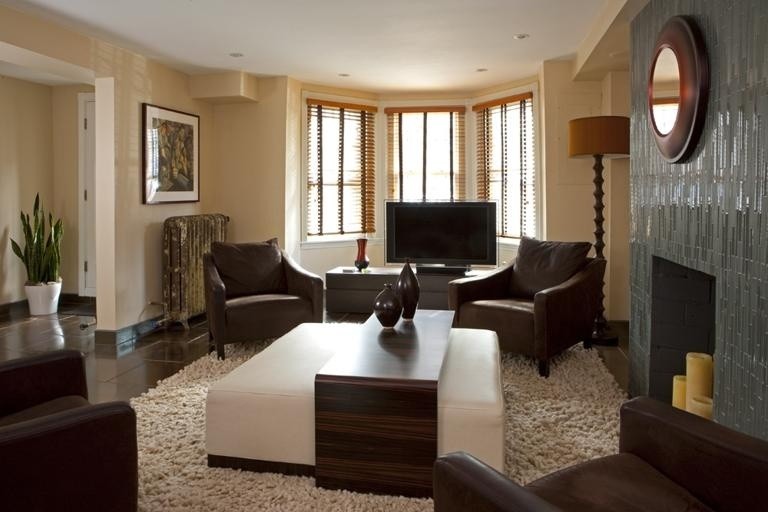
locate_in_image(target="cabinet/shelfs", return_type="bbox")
[326,265,507,314]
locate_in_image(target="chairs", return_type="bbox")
[433,396,767,512]
[205,237,323,360]
[449,257,602,377]
[0,349,141,512]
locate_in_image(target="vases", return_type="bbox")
[394,259,421,321]
[372,284,403,329]
[353,238,370,273]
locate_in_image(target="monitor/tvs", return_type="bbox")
[383,198,498,275]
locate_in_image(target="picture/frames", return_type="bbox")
[140,103,202,205]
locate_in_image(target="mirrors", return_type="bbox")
[647,14,710,165]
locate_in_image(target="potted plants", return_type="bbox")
[8,189,66,317]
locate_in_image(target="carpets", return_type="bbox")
[127,340,632,512]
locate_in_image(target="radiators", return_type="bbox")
[136,213,231,334]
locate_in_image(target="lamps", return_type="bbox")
[566,116,629,349]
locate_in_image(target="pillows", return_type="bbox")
[509,236,593,302]
[211,237,285,298]
